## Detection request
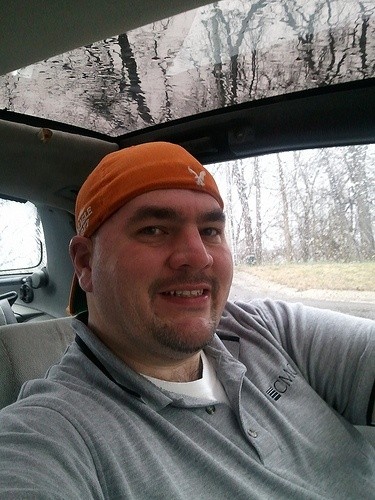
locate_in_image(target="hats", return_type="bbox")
[68,142,224,317]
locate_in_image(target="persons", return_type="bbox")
[0,141,375,500]
